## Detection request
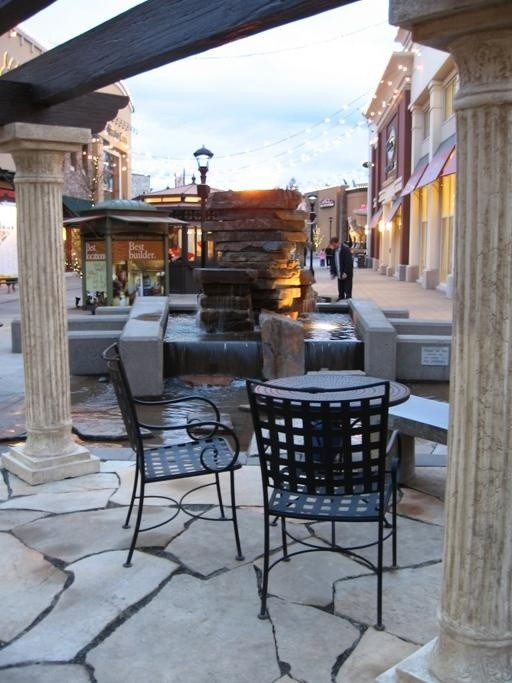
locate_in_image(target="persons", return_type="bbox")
[319,245,332,267]
[113,270,128,297]
[328,236,353,301]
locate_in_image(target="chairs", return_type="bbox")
[106,342,246,567]
[245,378,400,629]
[303,341,364,378]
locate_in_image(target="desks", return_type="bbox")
[255,376,410,528]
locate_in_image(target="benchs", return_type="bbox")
[388,394,450,483]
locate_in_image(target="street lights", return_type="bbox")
[194,142,213,265]
[328,215,334,244]
[307,192,318,274]
